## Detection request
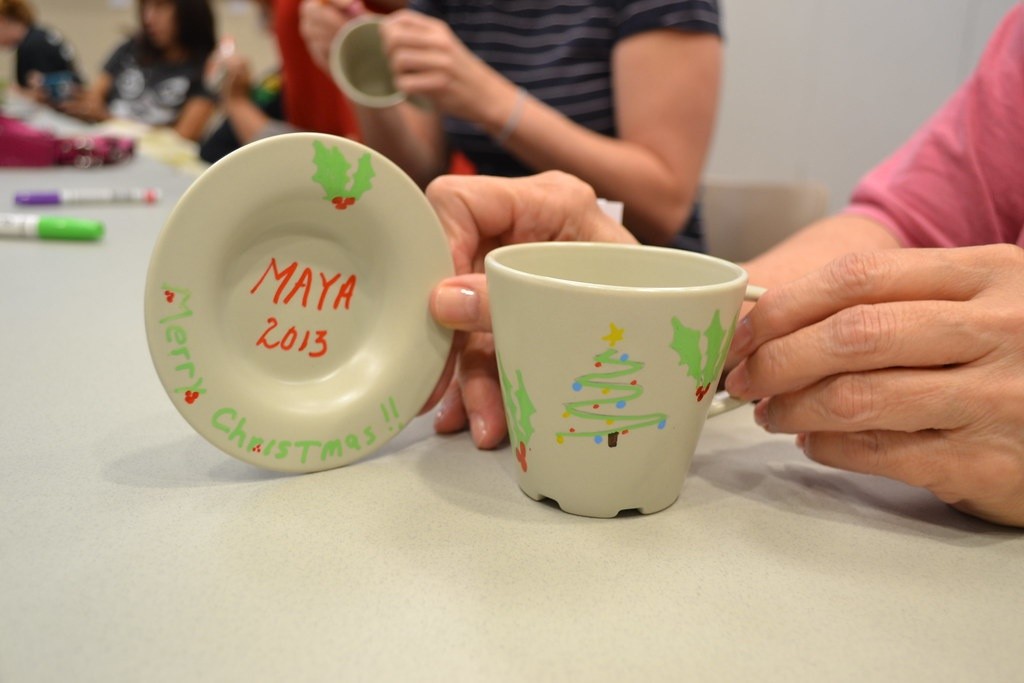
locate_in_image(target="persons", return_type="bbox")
[0,0,474,177]
[301,0,726,254]
[415,0,1024,531]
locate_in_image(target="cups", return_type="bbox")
[484,241,769,518]
[330,14,414,108]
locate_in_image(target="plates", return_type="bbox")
[143,131,458,475]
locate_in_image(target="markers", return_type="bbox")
[1,213,104,241]
[14,190,155,204]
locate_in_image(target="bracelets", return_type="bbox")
[497,88,528,146]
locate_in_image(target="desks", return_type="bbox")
[0,155,1024,683]
